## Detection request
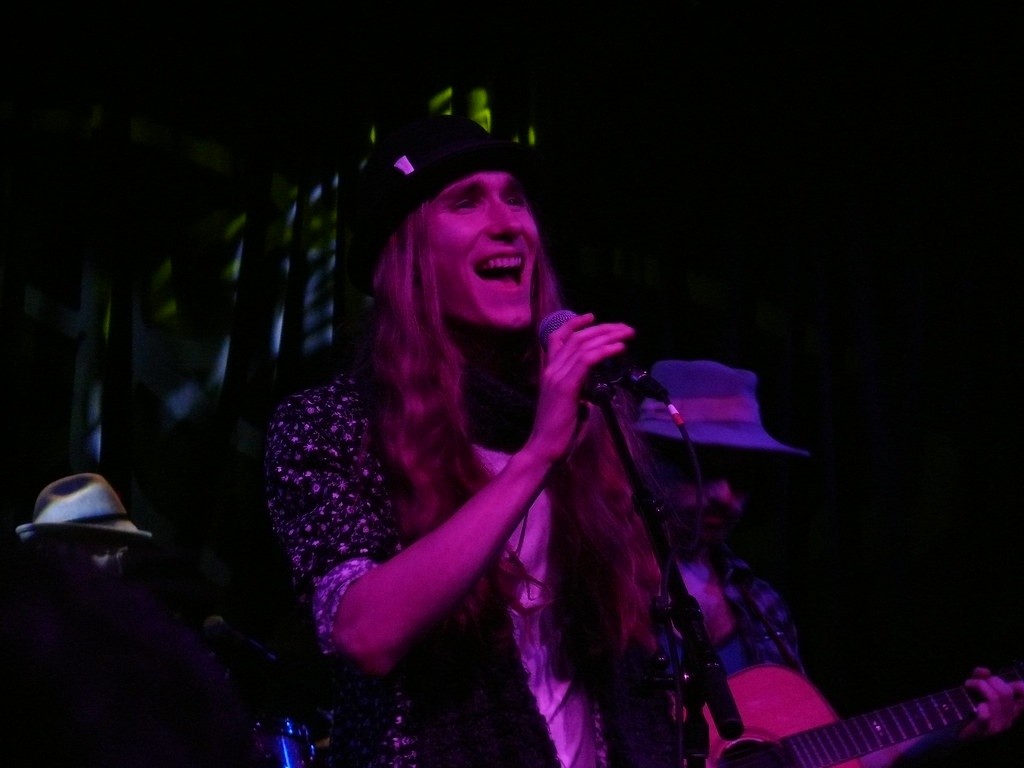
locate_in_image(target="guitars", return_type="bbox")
[662,662,1024,768]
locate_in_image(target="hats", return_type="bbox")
[355,113,536,247]
[638,359,810,457]
[14,470,155,543]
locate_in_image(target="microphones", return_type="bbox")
[540,310,673,403]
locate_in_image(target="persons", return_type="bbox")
[637,361,1023,768]
[16,474,229,684]
[265,114,684,766]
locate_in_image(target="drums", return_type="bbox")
[253,719,315,768]
